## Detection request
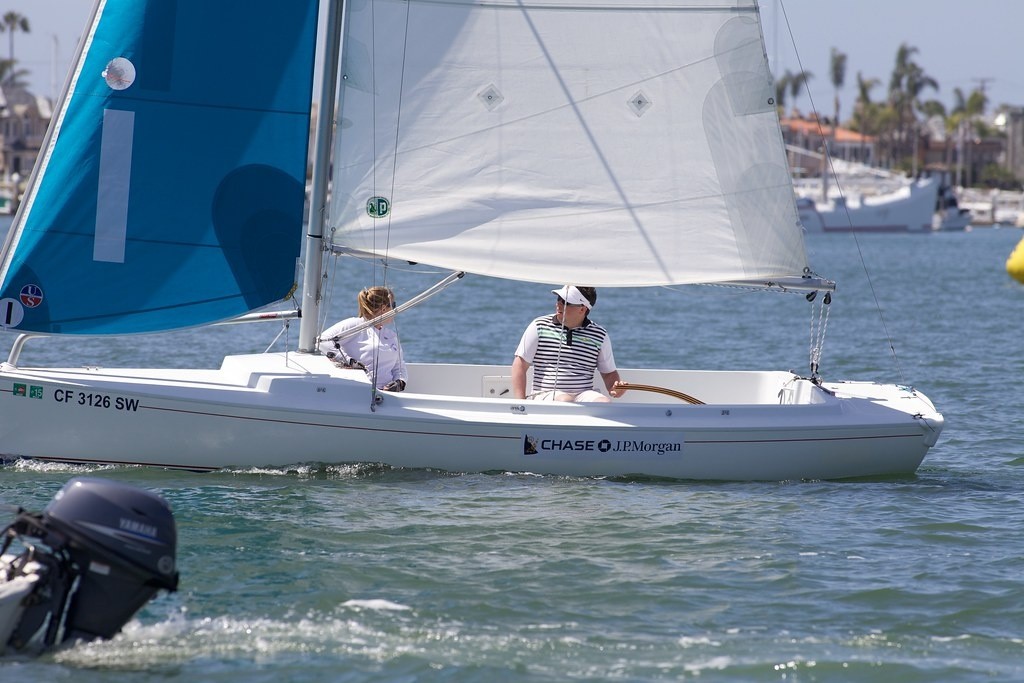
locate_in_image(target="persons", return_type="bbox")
[315,286,408,392]
[512,286,629,403]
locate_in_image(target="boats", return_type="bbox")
[792,142,973,233]
[0,476,178,661]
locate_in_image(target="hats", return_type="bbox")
[551,285,593,310]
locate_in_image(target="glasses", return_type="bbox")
[557,297,584,308]
[385,302,396,309]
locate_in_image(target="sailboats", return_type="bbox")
[0,0,942,483]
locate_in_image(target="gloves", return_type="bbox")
[350,359,369,374]
